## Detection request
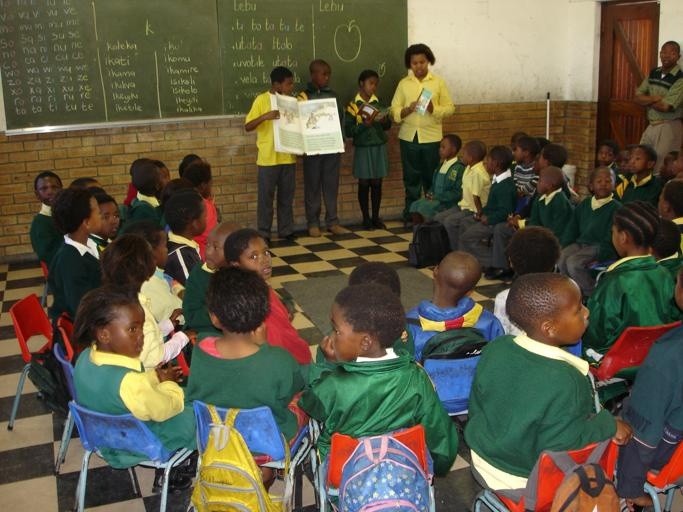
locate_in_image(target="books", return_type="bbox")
[270,91,346,155]
[414,86,433,117]
[358,103,392,126]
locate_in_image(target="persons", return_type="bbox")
[632,41,683,176]
[295,58,353,238]
[244,65,298,246]
[344,69,392,229]
[388,44,456,220]
[28,132,682,510]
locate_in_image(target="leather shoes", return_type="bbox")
[487,269,514,280]
[152,469,192,494]
[172,464,196,477]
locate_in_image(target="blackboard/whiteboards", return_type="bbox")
[0,0,409,137]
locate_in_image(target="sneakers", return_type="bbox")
[308,226,321,237]
[266,238,273,247]
[327,224,353,235]
[279,235,297,240]
[373,220,386,230]
[364,220,375,231]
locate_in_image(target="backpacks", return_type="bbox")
[322,428,433,512]
[408,221,450,268]
[187,404,293,512]
[28,348,72,419]
[406,327,487,366]
[523,439,620,512]
